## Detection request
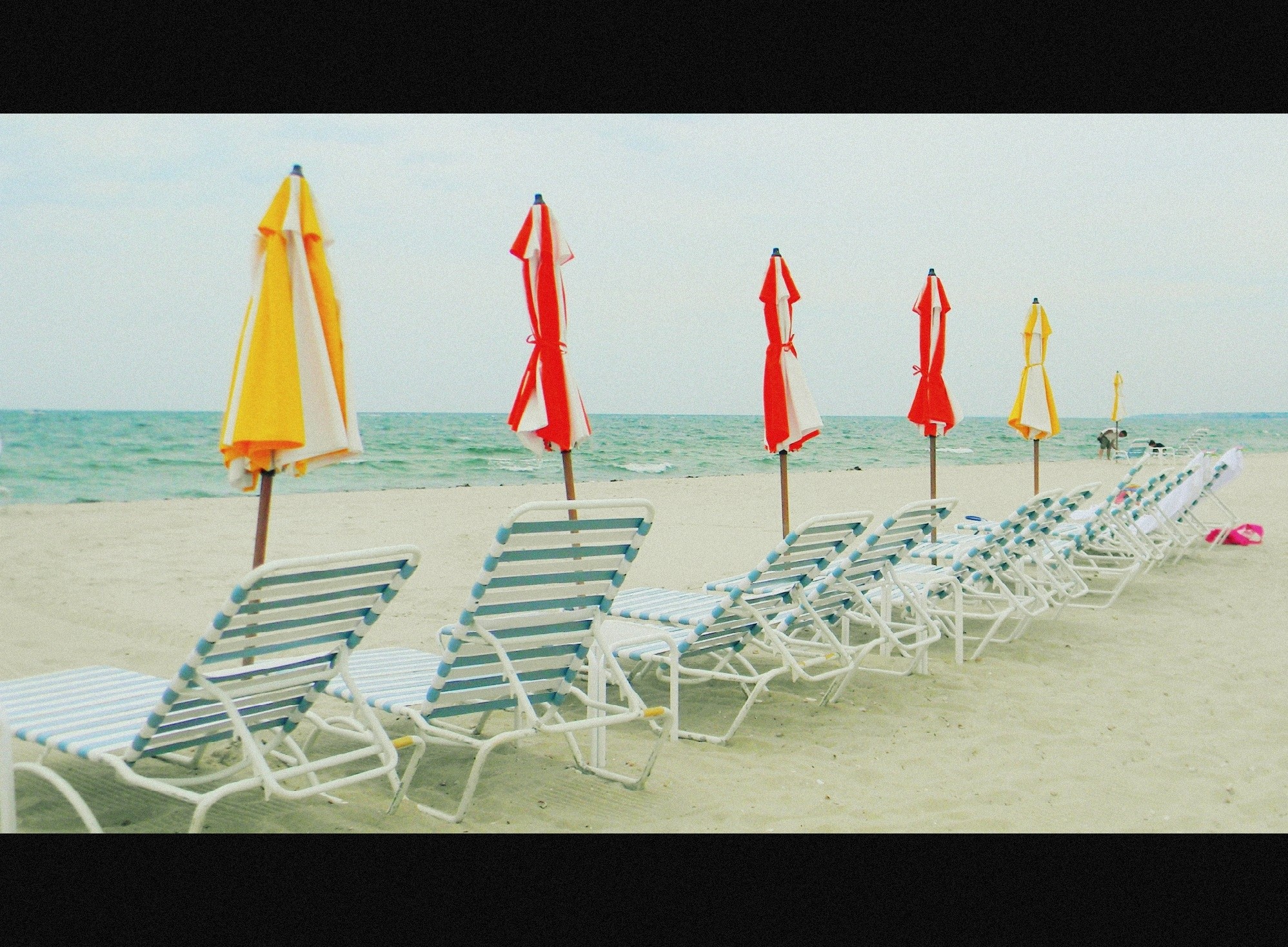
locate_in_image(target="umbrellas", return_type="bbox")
[759,248,824,570]
[505,194,593,664]
[1112,371,1128,463]
[1007,298,1061,495]
[908,268,965,564]
[204,160,363,763]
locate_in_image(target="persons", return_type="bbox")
[1149,439,1167,452]
[1098,427,1127,460]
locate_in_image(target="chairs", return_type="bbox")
[0,442,1251,836]
